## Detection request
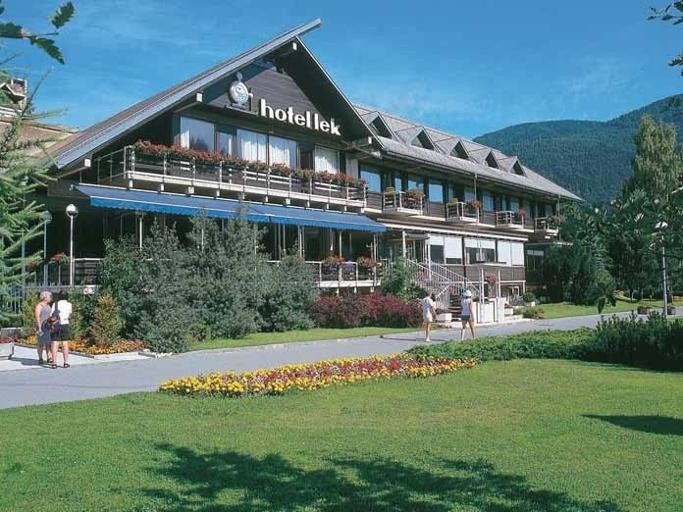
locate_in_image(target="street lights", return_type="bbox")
[656,222,670,312]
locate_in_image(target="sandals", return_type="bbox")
[47,359,57,369]
[39,359,43,364]
[64,364,70,368]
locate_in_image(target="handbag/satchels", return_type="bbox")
[46,311,60,333]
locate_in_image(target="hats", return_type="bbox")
[463,290,472,296]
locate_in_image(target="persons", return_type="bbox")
[421,289,437,343]
[32,291,53,366]
[47,291,72,368]
[458,290,478,343]
